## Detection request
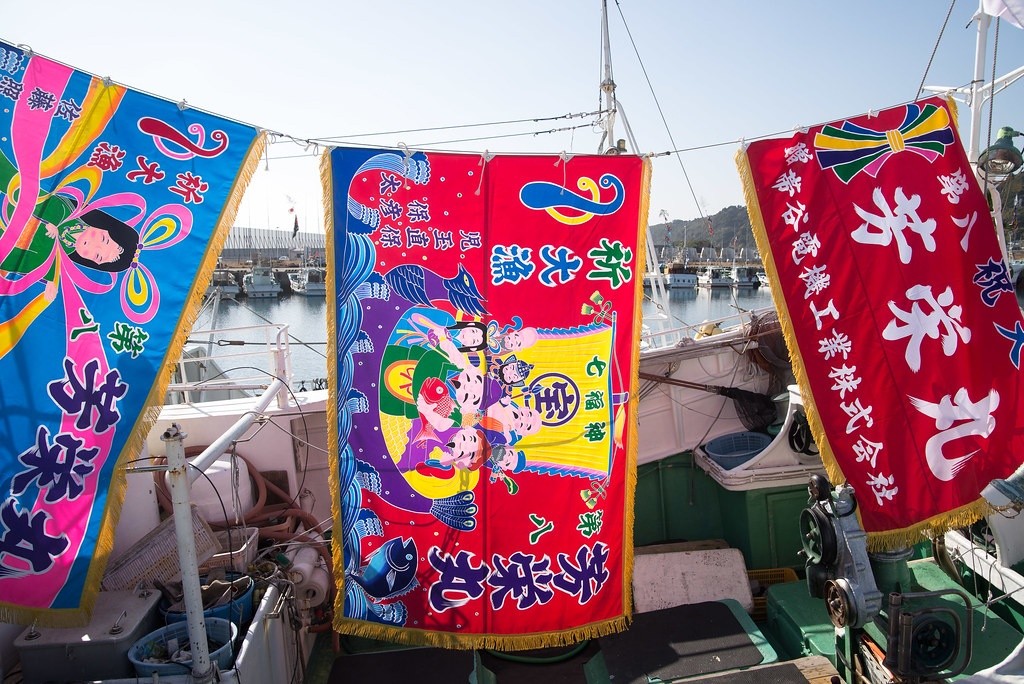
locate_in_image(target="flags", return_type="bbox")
[292,215,299,238]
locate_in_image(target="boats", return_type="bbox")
[204,210,769,302]
